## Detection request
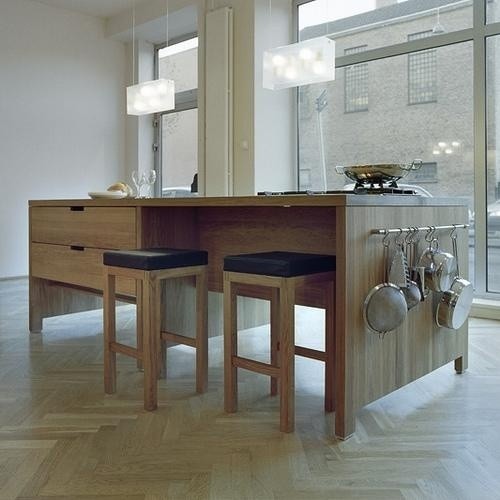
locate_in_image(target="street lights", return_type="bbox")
[432,140,462,195]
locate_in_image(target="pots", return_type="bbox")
[418,235,474,330]
[335,159,424,183]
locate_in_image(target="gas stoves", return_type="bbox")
[257,183,422,198]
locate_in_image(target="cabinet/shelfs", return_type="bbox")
[30,199,145,300]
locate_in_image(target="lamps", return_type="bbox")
[433,140,461,157]
[263,0,335,91]
[126,0,175,116]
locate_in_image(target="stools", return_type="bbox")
[222,251,336,433]
[102,248,209,411]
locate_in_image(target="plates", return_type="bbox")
[87,190,128,199]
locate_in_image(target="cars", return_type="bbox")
[340,184,474,229]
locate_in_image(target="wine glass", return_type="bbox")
[132,170,156,199]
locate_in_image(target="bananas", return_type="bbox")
[107,182,128,191]
